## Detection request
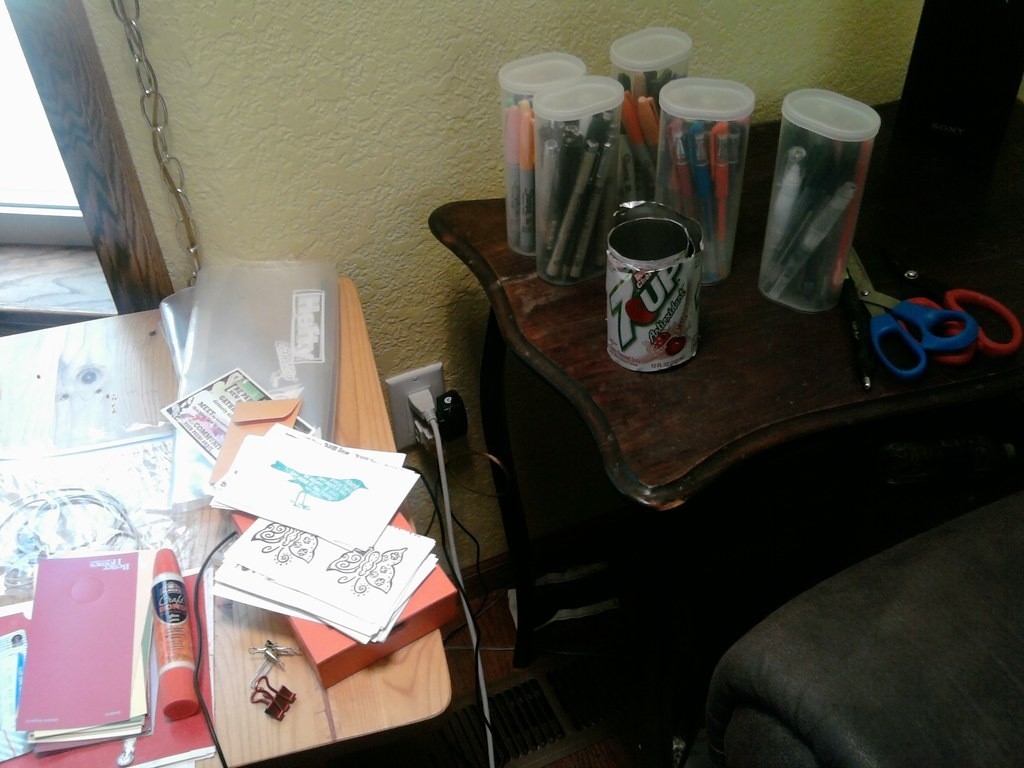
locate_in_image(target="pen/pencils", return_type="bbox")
[758,90,876,318]
[655,79,751,286]
[840,270,873,389]
[501,27,685,283]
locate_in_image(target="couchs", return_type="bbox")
[705,493,1024,768]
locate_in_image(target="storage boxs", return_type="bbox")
[228,513,463,693]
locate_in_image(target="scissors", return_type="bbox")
[845,247,1022,381]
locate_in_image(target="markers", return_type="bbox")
[149,548,202,720]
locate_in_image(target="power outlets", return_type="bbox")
[385,362,446,451]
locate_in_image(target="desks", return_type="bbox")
[426,84,1024,768]
[0,282,452,768]
